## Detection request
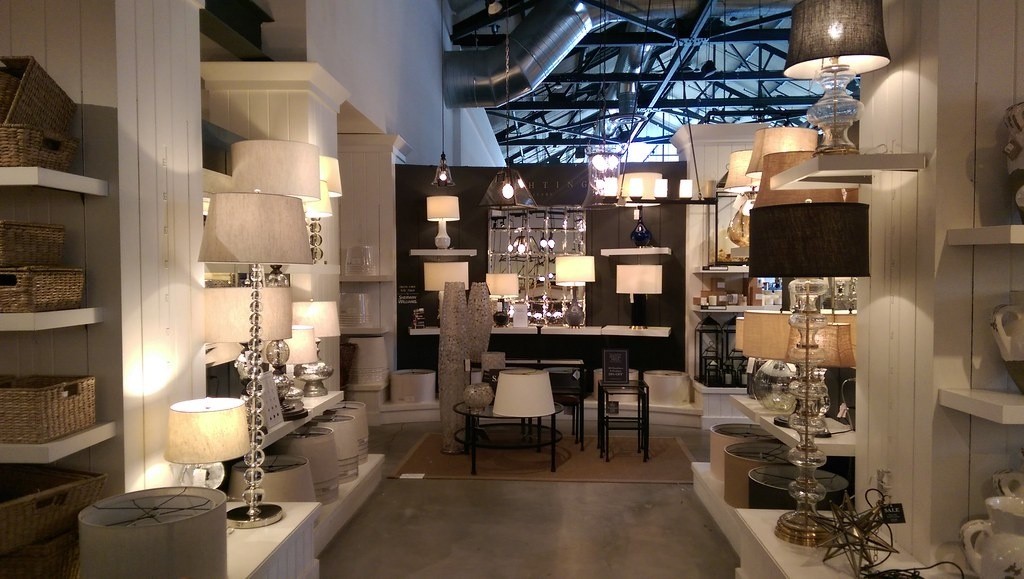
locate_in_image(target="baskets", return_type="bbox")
[0,56,77,134]
[0,219,64,266]
[0,528,82,579]
[0,267,84,313]
[0,462,108,554]
[0,376,96,444]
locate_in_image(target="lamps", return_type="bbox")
[78,139,343,579]
[425,1,899,579]
[389,368,436,403]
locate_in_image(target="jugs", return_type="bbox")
[958,443,1024,579]
[988,289,1024,394]
[1000,100,1024,220]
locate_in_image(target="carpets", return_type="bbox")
[388,430,698,484]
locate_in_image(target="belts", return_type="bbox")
[0,123,80,171]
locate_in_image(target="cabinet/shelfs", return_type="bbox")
[452,400,565,478]
[598,380,650,462]
[202,263,385,558]
[770,152,1024,425]
[692,267,856,459]
[0,165,116,464]
[338,275,390,426]
[407,247,672,337]
